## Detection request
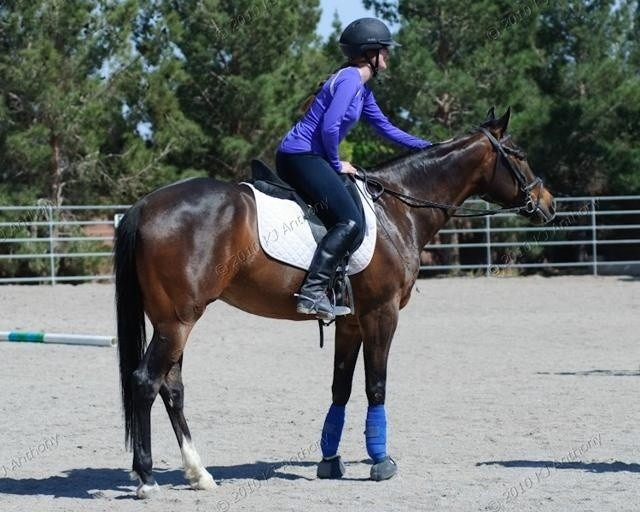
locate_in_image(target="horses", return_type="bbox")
[106,103,560,495]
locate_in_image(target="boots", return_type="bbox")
[296,215,362,318]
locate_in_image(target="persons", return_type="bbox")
[272,16,433,318]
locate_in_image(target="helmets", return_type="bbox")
[339,16,402,61]
